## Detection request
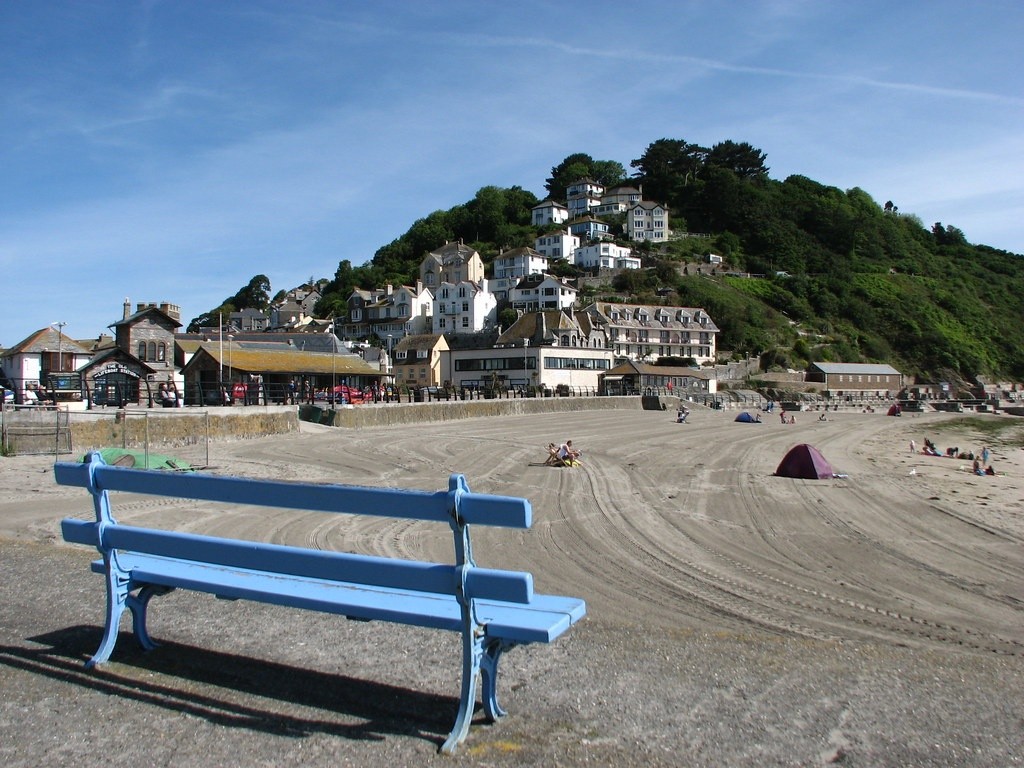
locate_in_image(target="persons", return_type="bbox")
[222,380,475,404]
[755,400,827,424]
[161,384,181,408]
[909,436,995,476]
[549,440,579,467]
[26,384,52,411]
[667,381,689,424]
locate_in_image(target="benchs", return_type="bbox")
[54,451,586,755]
[153,393,185,404]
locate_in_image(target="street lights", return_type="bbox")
[52,321,69,371]
[227,334,235,378]
[524,338,529,384]
[388,334,392,383]
[746,351,748,380]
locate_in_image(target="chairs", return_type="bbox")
[544,443,568,467]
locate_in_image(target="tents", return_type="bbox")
[734,412,755,423]
[772,443,833,479]
[887,404,901,416]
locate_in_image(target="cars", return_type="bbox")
[329,386,364,404]
